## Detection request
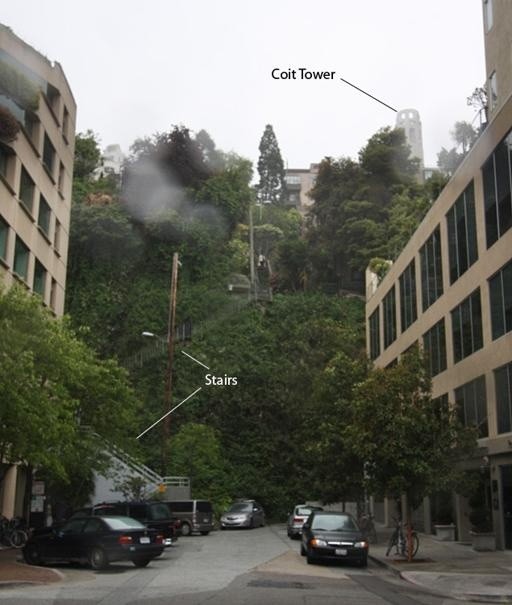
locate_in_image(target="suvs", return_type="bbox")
[69,501,178,546]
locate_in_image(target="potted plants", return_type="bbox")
[433,490,496,552]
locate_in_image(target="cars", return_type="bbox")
[286,504,323,539]
[23,515,165,570]
[300,510,368,568]
[219,502,265,529]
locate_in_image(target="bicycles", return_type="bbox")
[357,506,376,537]
[0,515,35,549]
[385,517,419,558]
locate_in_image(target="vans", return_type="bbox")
[161,499,213,536]
